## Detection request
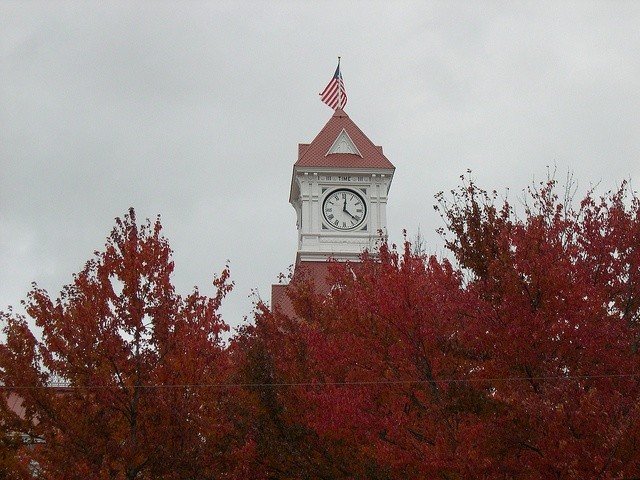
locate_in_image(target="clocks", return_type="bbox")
[317,182,372,234]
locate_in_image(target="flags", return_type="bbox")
[318,57,348,111]
